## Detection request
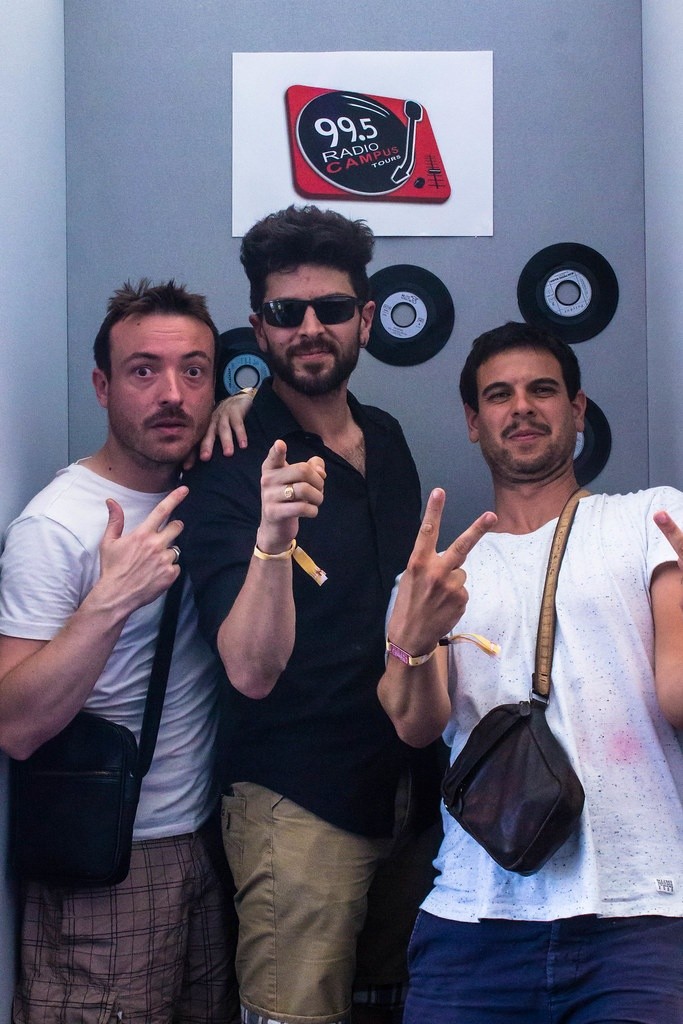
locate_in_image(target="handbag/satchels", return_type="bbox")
[7,710,142,889]
[440,703,585,876]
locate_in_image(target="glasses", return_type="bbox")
[256,295,366,329]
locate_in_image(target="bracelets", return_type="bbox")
[232,386,258,399]
[254,538,328,586]
[385,631,502,667]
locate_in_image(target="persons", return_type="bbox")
[0,205,446,1024]
[376,323,683,1024]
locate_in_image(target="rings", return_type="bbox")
[167,545,182,564]
[284,483,296,501]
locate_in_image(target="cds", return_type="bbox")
[362,265,455,367]
[572,397,612,487]
[517,241,618,344]
[213,326,272,405]
